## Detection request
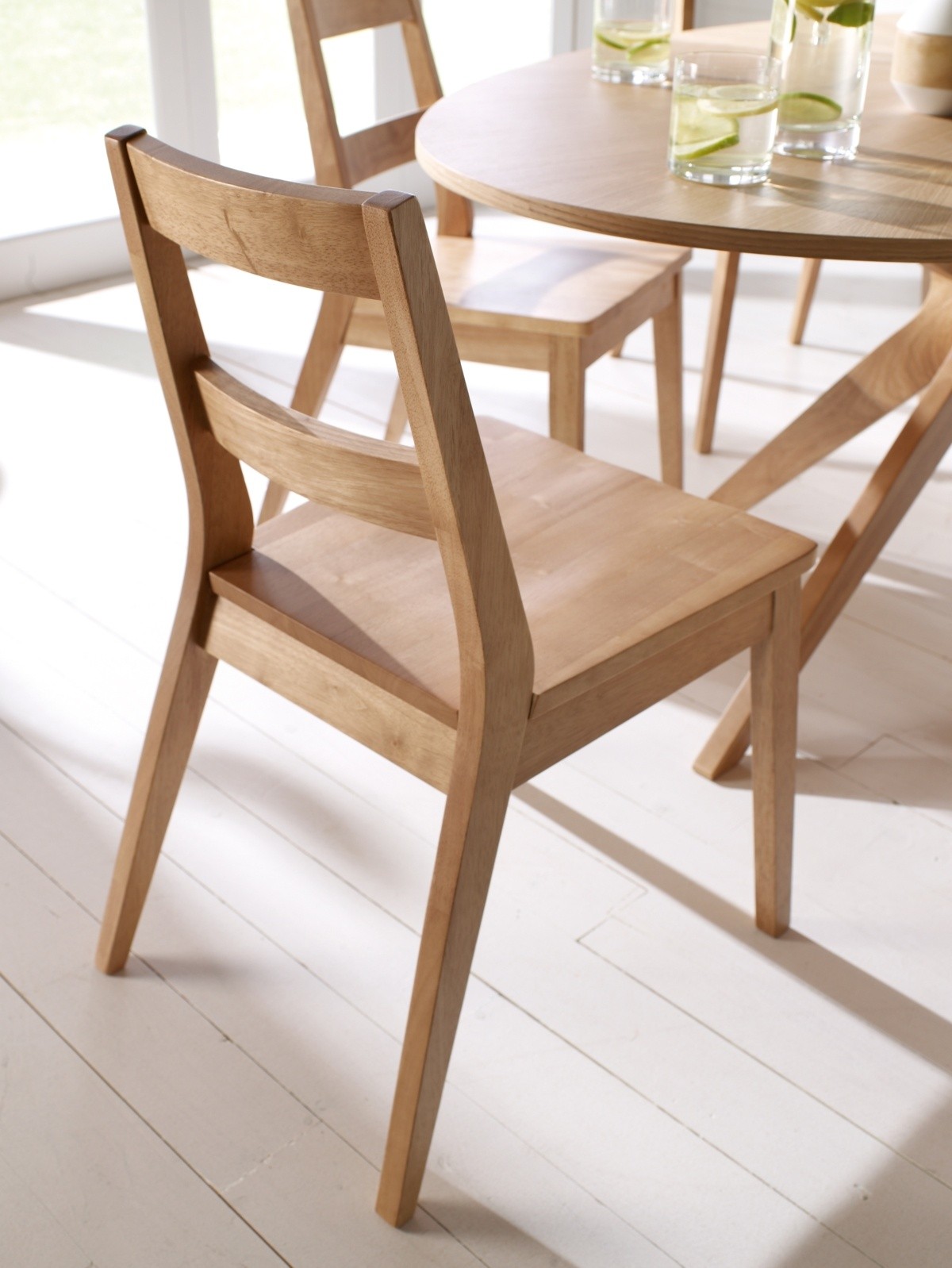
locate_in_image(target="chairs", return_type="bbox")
[92,123,822,1231]
[258,0,693,525]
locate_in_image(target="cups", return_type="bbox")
[668,52,780,187]
[764,0,876,160]
[891,0,952,114]
[590,0,684,86]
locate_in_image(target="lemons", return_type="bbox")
[786,0,875,41]
[593,31,669,58]
[671,96,779,159]
[777,91,841,123]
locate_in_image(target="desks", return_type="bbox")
[412,14,952,781]
